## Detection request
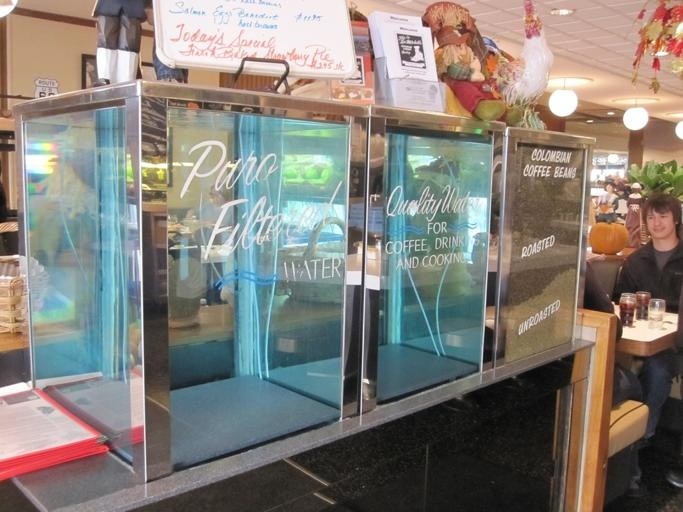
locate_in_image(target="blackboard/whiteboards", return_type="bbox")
[152,0,359,79]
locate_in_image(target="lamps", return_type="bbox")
[549,76,592,118]
[670,112,683,139]
[612,97,658,132]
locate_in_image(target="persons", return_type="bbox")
[185,183,234,306]
[612,193,683,488]
[624,182,642,248]
[592,178,620,223]
[583,260,645,410]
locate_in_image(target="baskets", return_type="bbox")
[0,255,50,336]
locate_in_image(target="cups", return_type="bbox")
[637,290,652,319]
[620,292,637,326]
[648,299,666,328]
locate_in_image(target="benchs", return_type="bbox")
[486,305,649,512]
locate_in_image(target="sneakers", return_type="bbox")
[628,464,682,499]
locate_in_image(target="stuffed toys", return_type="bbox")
[422,2,555,125]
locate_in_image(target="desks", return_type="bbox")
[614,304,678,358]
